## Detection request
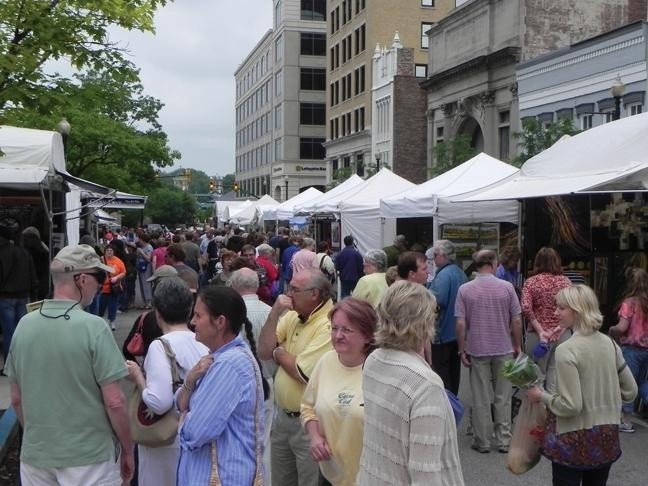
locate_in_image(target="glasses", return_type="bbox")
[84,270,108,285]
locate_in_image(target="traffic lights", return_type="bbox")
[234,181,238,192]
[209,181,216,194]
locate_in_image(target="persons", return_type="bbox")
[0,215,648,485]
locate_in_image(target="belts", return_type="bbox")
[286,410,301,417]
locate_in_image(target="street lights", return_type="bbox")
[609,71,624,122]
[58,115,72,163]
[262,179,267,194]
[283,175,289,200]
[374,148,382,172]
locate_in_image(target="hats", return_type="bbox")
[146,265,177,282]
[51,244,115,275]
[125,241,136,249]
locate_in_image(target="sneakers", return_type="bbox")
[618,423,635,434]
[499,446,508,453]
[471,441,490,453]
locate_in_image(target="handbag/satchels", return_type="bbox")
[110,284,123,296]
[127,337,182,447]
[319,256,335,284]
[126,312,148,355]
